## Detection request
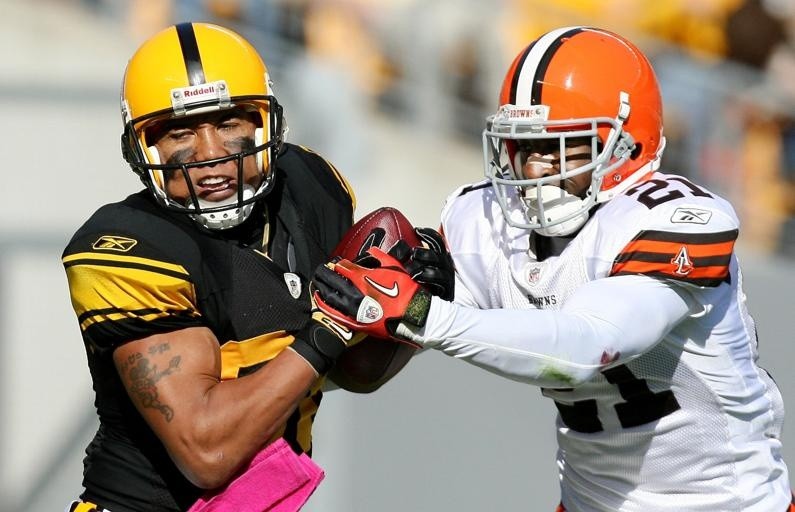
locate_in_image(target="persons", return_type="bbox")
[60,19,457,512]
[310,24,794,512]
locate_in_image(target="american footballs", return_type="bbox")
[326,207,429,393]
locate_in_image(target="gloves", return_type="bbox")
[290,226,456,374]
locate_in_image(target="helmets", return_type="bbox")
[120,22,288,229]
[481,26,667,237]
